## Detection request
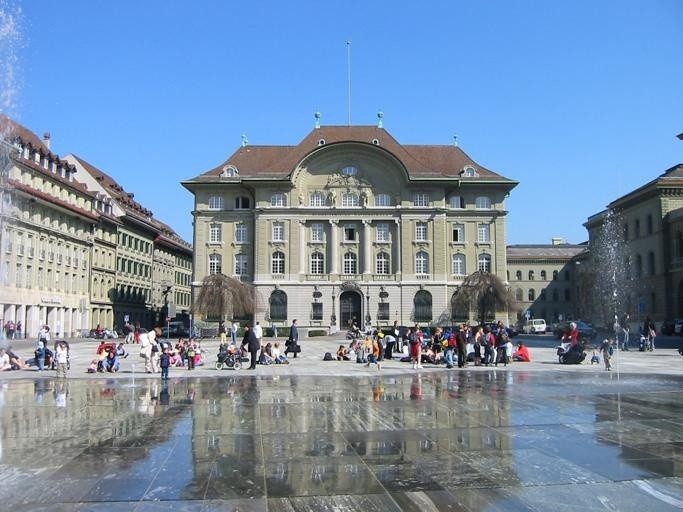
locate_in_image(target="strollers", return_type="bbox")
[51,340,70,370]
[215,348,246,370]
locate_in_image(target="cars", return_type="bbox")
[553,321,597,341]
[523,319,547,334]
[660,318,683,335]
[472,323,519,337]
[160,322,190,338]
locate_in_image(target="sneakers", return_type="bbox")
[285,351,297,357]
[413,364,423,368]
[606,368,613,371]
[485,363,508,367]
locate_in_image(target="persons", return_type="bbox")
[136,378,266,416]
[560,312,656,371]
[0,317,298,380]
[336,317,530,369]
[368,371,513,402]
[54,382,71,408]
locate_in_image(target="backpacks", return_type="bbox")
[480,335,488,346]
[408,334,418,345]
[498,331,508,344]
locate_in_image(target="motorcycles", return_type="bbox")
[556,336,587,363]
[346,328,362,340]
[639,332,650,351]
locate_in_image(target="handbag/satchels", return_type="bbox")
[289,345,301,352]
